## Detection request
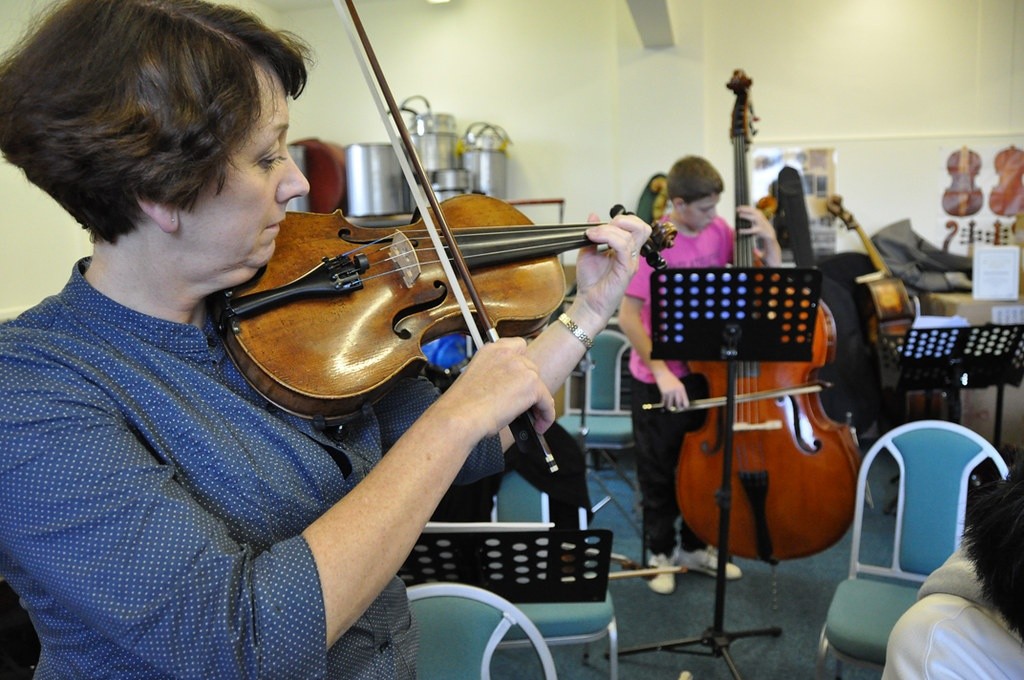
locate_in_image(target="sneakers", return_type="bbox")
[678,547,742,581]
[648,553,676,594]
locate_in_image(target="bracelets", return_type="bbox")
[557,310,595,352]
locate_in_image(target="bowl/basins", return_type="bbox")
[434,190,464,204]
[431,168,469,191]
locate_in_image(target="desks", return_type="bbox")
[923,293,1024,448]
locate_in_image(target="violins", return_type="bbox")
[211,194,680,428]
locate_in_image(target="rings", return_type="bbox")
[629,251,638,258]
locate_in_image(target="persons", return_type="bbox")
[883,463,1024,680]
[0,0,653,680]
[617,157,782,596]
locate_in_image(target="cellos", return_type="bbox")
[673,66,863,610]
[826,194,947,438]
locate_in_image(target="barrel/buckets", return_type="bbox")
[401,132,458,213]
[461,149,507,200]
[344,142,404,217]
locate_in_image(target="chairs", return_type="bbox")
[407,330,635,680]
[816,420,1009,679]
